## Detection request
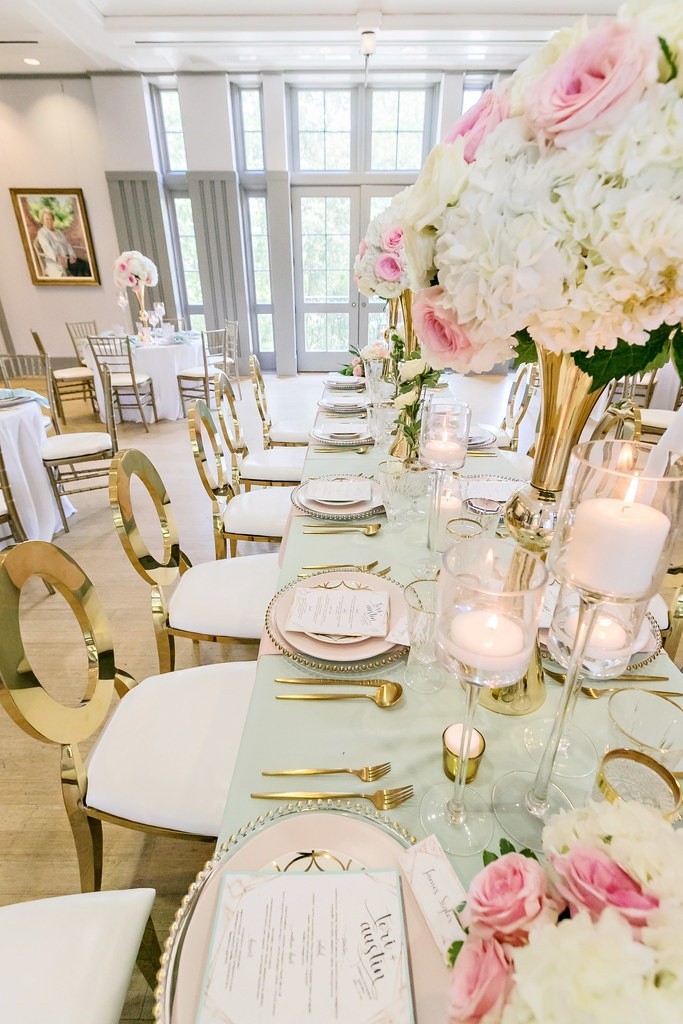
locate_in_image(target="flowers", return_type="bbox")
[354,205,410,299]
[112,249,158,294]
[395,359,449,461]
[399,0,683,394]
[440,796,683,1023]
[362,338,393,361]
[339,344,365,378]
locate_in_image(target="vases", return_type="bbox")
[389,286,422,462]
[503,334,608,553]
[134,292,152,342]
[382,296,401,383]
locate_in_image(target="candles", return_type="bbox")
[564,608,626,661]
[450,611,525,669]
[426,428,460,463]
[563,469,671,595]
[445,724,479,778]
[429,489,461,552]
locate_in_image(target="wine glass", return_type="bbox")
[364,357,397,455]
[403,578,446,694]
[377,458,430,528]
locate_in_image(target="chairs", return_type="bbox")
[249,353,315,447]
[85,335,159,433]
[601,367,659,410]
[188,399,300,561]
[29,329,100,426]
[0,886,164,1024]
[176,325,228,419]
[673,384,683,412]
[108,447,280,676]
[639,406,679,434]
[208,318,244,400]
[65,320,98,403]
[0,445,57,598]
[39,361,119,533]
[498,363,537,453]
[0,540,259,894]
[569,397,642,514]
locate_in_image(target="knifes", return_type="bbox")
[274,678,391,687]
[303,522,377,528]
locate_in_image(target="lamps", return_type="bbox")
[356,8,385,92]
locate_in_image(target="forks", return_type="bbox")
[542,667,669,682]
[296,567,391,577]
[302,561,378,570]
[580,686,683,699]
[261,761,391,783]
[251,785,414,811]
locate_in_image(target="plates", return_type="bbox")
[0,396,25,406]
[467,425,498,450]
[461,474,526,505]
[290,473,384,521]
[317,394,367,412]
[309,422,374,446]
[323,376,365,389]
[264,568,422,676]
[151,801,450,1024]
[540,608,662,675]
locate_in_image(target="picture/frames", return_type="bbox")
[9,187,101,287]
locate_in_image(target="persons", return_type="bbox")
[38,209,77,278]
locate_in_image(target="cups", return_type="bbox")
[588,747,683,818]
[604,687,683,769]
[442,725,485,783]
[133,322,174,345]
[461,498,504,538]
[444,518,482,552]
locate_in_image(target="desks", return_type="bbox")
[200,367,683,886]
[0,387,78,552]
[82,326,211,425]
[211,367,683,900]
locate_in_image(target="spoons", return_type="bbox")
[303,524,381,535]
[275,682,403,708]
[313,445,369,454]
